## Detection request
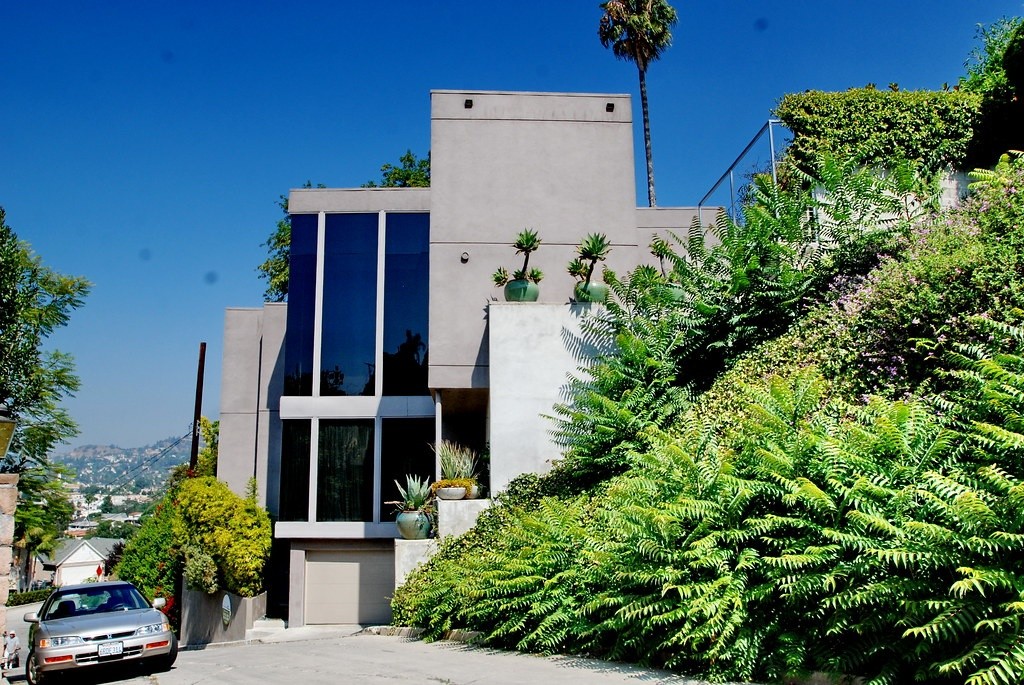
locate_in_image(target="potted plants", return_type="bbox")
[566,230,612,302]
[426,439,485,500]
[384,473,435,539]
[491,227,544,302]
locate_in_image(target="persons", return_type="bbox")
[4,630,19,669]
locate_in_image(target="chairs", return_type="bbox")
[58,601,77,617]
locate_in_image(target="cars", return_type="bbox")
[23,581,178,685]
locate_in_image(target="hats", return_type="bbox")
[10,631,15,634]
